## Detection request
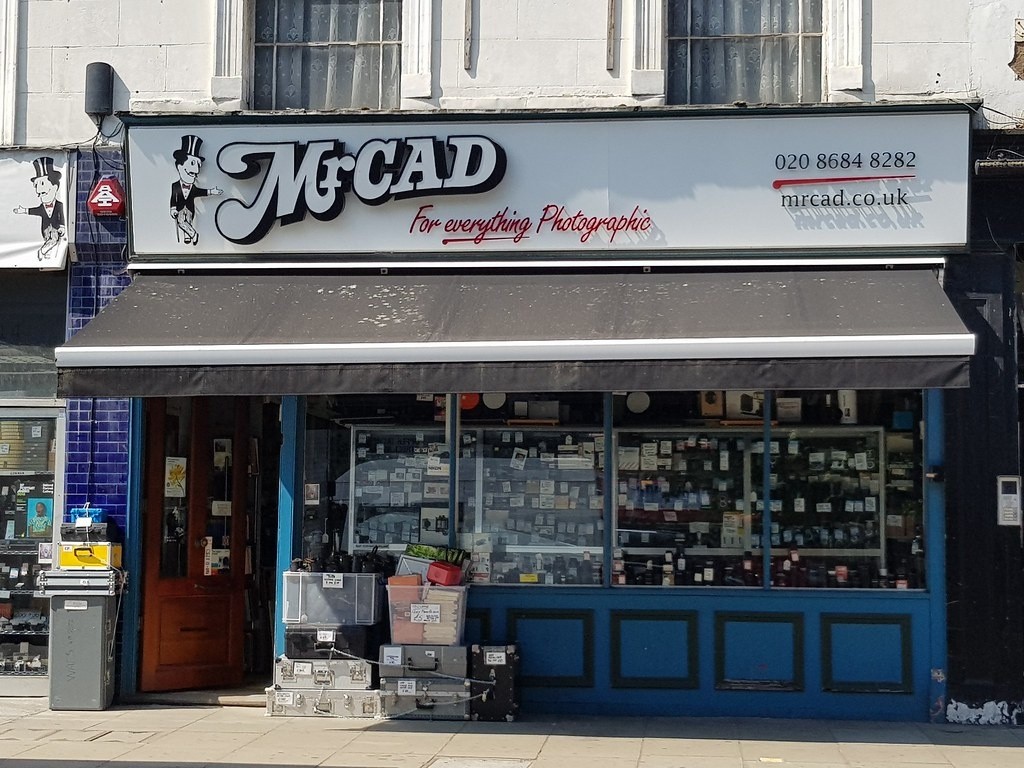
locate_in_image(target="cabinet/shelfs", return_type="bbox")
[348,423,888,592]
[0,536,52,696]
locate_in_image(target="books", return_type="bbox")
[423,589,460,642]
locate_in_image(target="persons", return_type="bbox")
[29,502,52,538]
[514,454,524,468]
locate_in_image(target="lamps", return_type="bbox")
[482,392,506,410]
[627,392,650,412]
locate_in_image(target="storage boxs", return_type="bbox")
[48,596,115,710]
[387,585,470,646]
[69,507,108,523]
[282,570,384,625]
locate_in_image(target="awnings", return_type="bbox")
[56,265,979,399]
